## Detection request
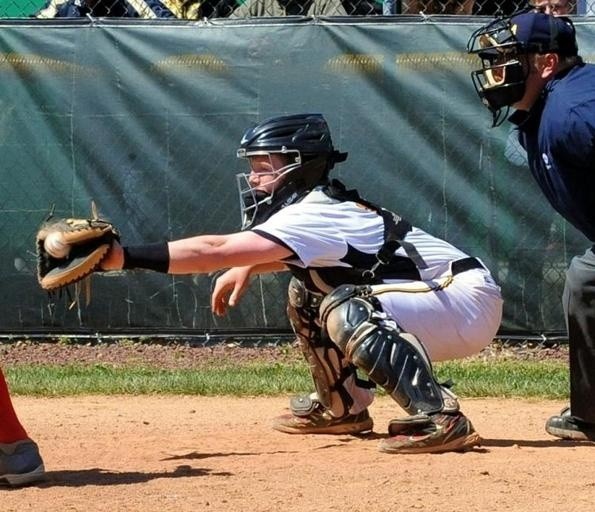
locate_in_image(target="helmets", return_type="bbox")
[234,113,332,233]
[465,10,579,131]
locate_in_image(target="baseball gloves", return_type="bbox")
[36,218,121,288]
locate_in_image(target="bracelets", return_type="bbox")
[122,242,170,276]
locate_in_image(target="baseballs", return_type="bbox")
[44,234,68,257]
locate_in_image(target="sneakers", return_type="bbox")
[543,408,594,441]
[0,438,45,486]
[377,413,480,454]
[272,395,376,435]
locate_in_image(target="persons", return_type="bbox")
[37,112,503,452]
[467,11,595,440]
[0,373,48,490]
